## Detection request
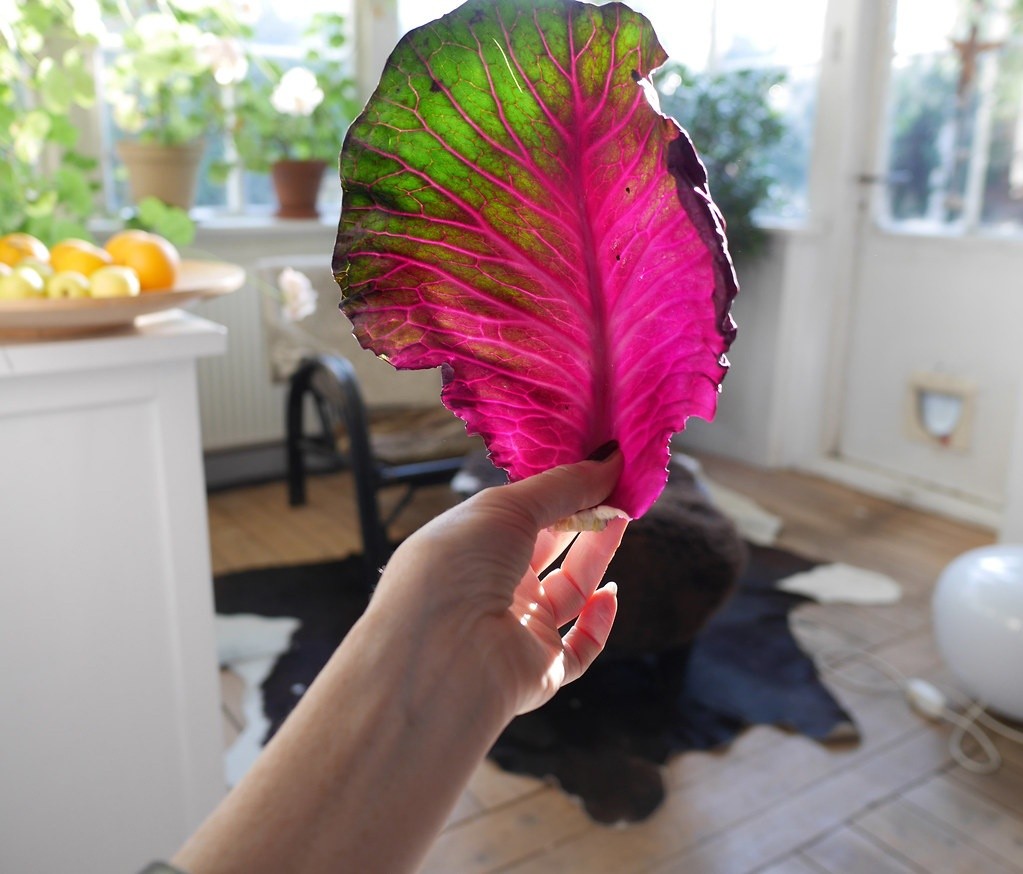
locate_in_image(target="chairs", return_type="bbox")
[258,254,488,560]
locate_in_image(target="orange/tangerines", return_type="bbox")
[0,229,182,292]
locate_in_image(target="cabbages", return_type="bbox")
[331,0,739,532]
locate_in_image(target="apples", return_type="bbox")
[0,263,141,300]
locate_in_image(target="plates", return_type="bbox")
[1,258,247,336]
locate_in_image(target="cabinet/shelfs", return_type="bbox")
[0,307,229,874]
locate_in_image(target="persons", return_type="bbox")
[132,438,630,874]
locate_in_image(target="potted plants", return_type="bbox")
[104,0,362,218]
